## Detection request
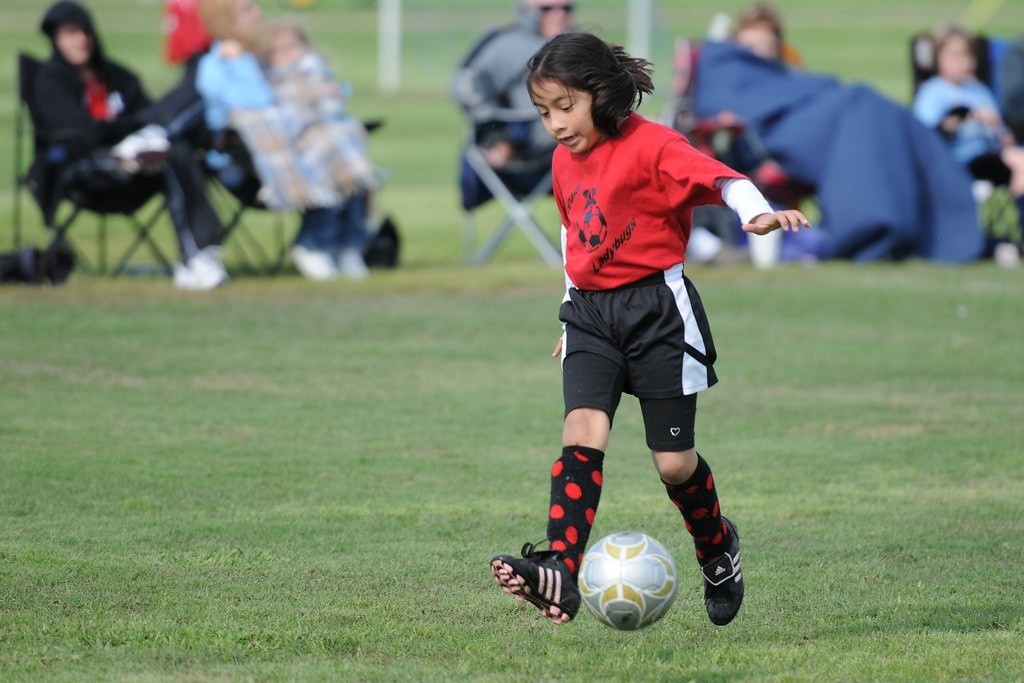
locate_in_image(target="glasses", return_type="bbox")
[540,5,572,13]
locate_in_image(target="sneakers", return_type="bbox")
[699,515,744,626]
[489,537,581,626]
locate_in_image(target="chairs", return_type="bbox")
[460,136,564,271]
[15,89,182,278]
[203,171,307,277]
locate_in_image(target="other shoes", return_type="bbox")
[173,250,227,291]
[342,247,371,276]
[293,243,338,282]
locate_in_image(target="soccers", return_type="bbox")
[577,531,679,632]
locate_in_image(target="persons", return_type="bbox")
[490,33,810,626]
[914,28,1024,267]
[195,0,380,277]
[673,1,821,225]
[462,0,575,176]
[31,1,227,290]
